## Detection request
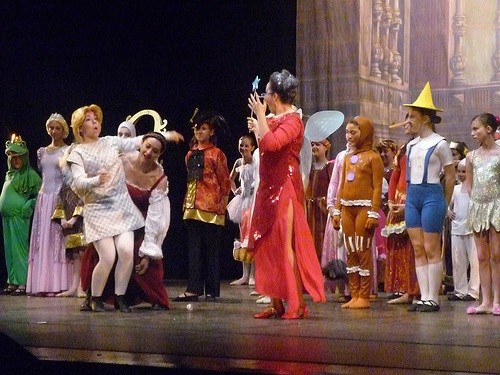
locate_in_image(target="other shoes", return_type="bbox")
[81,289,92,311]
[92,296,104,312]
[114,293,132,313]
[419,300,440,312]
[2,288,15,295]
[11,288,26,296]
[492,304,500,315]
[463,295,476,301]
[172,292,198,302]
[253,306,285,318]
[467,306,492,314]
[406,303,422,311]
[281,305,308,319]
[206,294,215,302]
[448,295,462,300]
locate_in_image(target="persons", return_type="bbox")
[230,69,500,320]
[57,104,184,314]
[0,133,43,297]
[174,111,230,298]
[25,113,85,297]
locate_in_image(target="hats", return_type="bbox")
[402,82,444,113]
